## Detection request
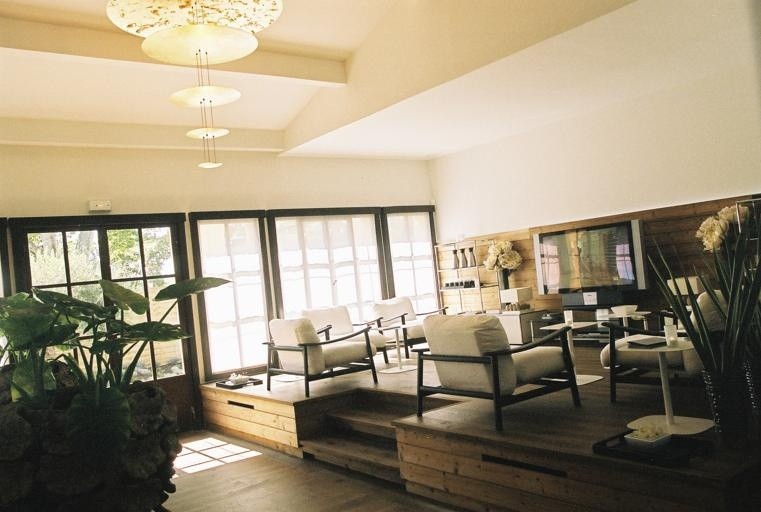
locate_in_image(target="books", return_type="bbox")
[627,338,667,350]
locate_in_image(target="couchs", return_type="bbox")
[302,295,448,374]
[262,318,378,397]
[600,288,735,403]
[412,313,581,430]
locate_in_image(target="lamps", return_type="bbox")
[106,1,283,170]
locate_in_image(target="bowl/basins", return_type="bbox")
[611,305,638,315]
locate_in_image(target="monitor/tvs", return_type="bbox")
[735,198,761,240]
[533,219,649,310]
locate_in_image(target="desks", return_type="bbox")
[599,311,651,338]
[539,322,604,386]
[617,340,716,436]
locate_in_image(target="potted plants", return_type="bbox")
[646,198,761,452]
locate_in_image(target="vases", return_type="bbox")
[497,272,510,308]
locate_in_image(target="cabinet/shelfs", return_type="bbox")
[434,240,499,313]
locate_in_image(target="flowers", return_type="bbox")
[483,241,523,272]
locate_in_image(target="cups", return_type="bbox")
[664,325,678,347]
[564,310,573,327]
